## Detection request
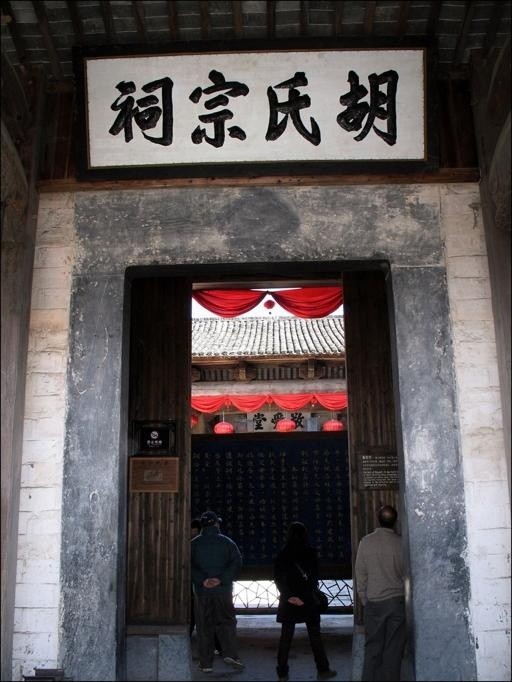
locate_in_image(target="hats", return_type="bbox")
[198,510,223,527]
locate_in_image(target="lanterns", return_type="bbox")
[191,414,197,429]
[276,418,295,432]
[214,421,233,435]
[264,300,275,314]
[323,419,344,432]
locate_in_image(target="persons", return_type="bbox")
[189,519,223,656]
[190,510,247,674]
[354,505,409,682]
[271,521,337,681]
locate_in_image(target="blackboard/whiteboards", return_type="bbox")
[190,432,353,580]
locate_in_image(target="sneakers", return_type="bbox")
[318,669,337,681]
[223,655,246,671]
[213,648,223,656]
[197,663,214,674]
[275,665,290,681]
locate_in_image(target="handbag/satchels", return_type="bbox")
[288,558,328,614]
